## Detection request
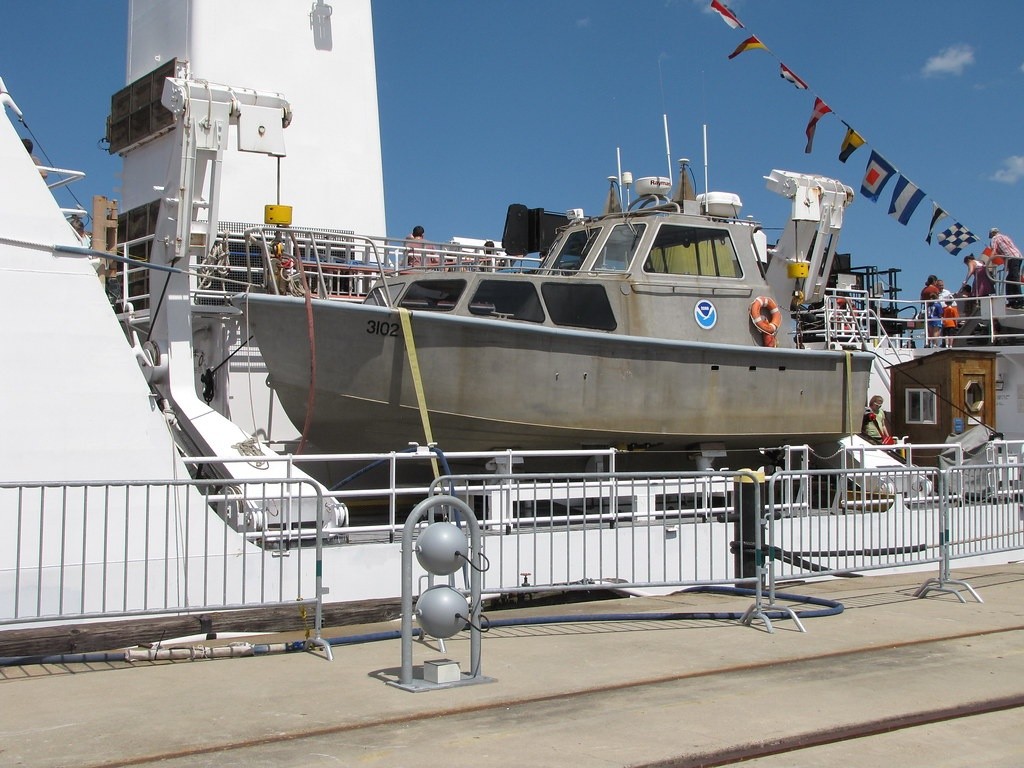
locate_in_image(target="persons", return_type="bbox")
[864,395,891,451]
[921,274,974,352]
[71,217,92,259]
[982,227,1024,307]
[962,253,997,296]
[479,240,500,273]
[404,226,439,270]
[20,139,49,182]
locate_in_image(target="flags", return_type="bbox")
[859,148,899,204]
[710,0,744,29]
[779,63,808,89]
[888,174,927,226]
[728,35,770,60]
[838,126,867,163]
[937,222,979,257]
[922,201,950,246]
[803,96,833,153]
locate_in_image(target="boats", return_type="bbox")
[225,115,873,475]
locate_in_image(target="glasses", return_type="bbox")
[874,402,881,407]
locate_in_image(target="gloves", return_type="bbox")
[869,413,876,421]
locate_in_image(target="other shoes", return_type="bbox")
[1006,298,1024,309]
[924,343,931,348]
[945,344,952,348]
[933,343,941,348]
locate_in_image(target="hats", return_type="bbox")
[988,228,999,238]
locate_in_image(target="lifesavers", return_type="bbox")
[751,295,781,333]
[832,299,859,331]
